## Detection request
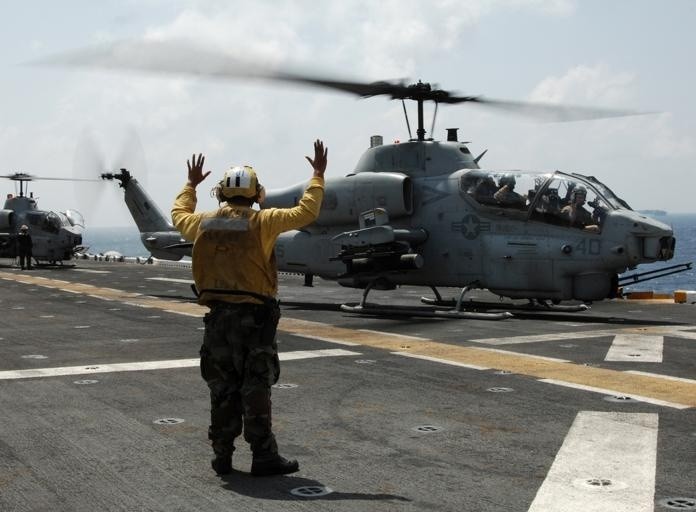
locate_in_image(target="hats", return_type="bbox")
[219,166,259,202]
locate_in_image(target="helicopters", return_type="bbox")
[0,173,111,270]
[13,36,685,323]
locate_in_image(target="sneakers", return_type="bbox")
[251,456,299,476]
[211,453,233,477]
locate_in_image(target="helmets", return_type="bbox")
[570,184,587,205]
[498,173,517,188]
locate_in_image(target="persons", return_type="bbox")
[169,139,328,477]
[14,223,34,270]
[559,184,601,233]
[493,172,527,209]
